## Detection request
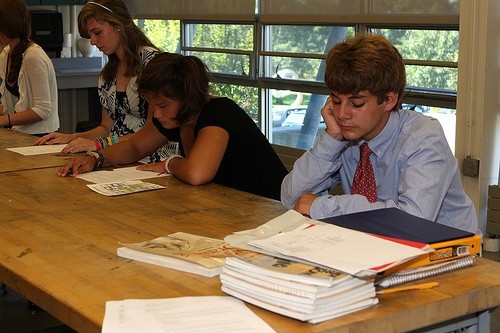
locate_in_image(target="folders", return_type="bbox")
[317,206,482,277]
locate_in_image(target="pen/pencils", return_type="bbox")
[376,281,441,295]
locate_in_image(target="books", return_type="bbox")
[117,231,249,278]
[220,208,481,324]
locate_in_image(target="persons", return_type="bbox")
[0,0,60,137]
[56,54,289,201]
[280,32,479,234]
[33,0,182,165]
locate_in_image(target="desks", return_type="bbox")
[0,126,76,172]
[0,167,500,333]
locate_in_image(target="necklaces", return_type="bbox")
[185,110,201,126]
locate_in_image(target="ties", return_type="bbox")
[351,142,377,204]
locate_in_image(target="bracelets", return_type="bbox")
[8,113,11,128]
[85,149,104,169]
[162,155,183,174]
[94,134,119,151]
